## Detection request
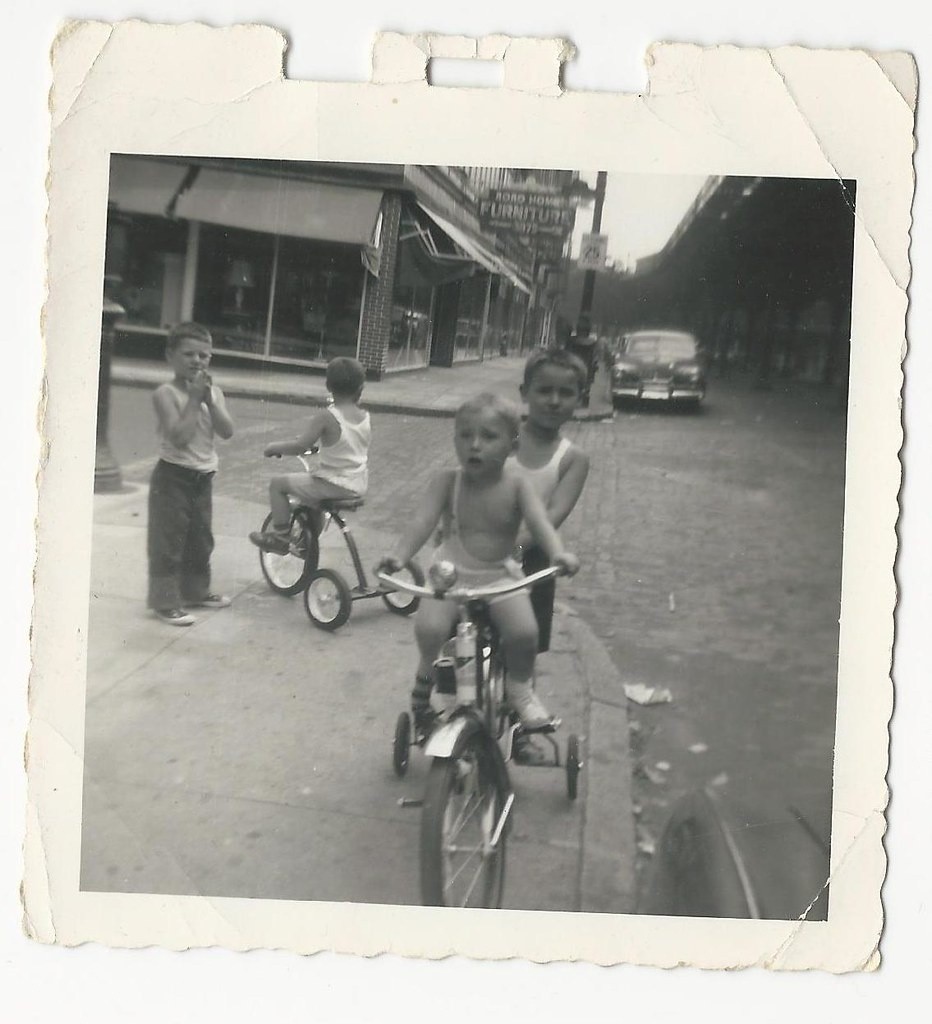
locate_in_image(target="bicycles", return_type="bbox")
[370,558,587,910]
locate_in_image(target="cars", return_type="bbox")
[598,326,709,413]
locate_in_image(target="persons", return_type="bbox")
[373,347,589,732]
[148,322,236,625]
[248,356,372,555]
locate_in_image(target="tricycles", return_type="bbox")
[251,441,429,633]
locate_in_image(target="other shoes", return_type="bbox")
[249,531,289,556]
[505,675,549,728]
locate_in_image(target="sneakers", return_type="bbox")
[153,606,195,624]
[184,593,229,607]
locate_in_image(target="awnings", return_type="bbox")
[173,166,386,247]
[412,200,502,277]
[109,156,189,220]
[474,240,532,295]
